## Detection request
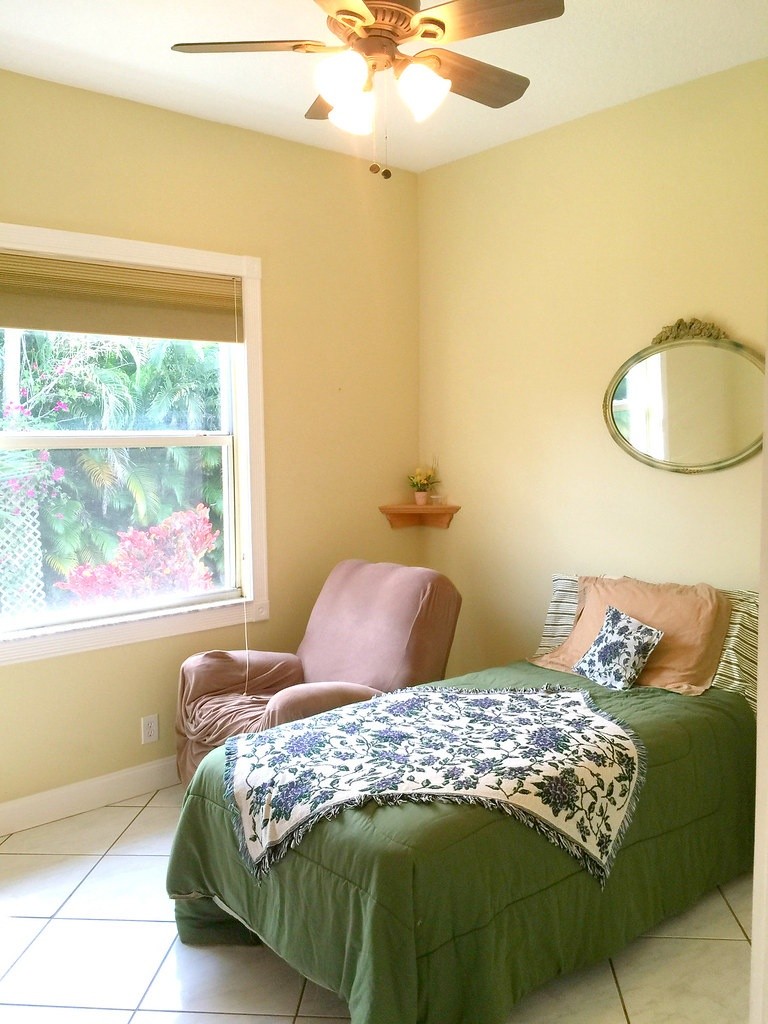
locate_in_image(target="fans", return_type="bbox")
[170,0,564,178]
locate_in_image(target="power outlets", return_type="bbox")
[141,713,158,744]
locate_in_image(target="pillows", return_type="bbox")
[525,574,733,697]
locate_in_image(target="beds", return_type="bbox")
[165,573,759,1024]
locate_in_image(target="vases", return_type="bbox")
[414,492,428,506]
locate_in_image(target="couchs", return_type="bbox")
[175,559,473,789]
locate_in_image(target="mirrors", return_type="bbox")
[602,319,765,473]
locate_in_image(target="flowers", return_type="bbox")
[408,457,441,493]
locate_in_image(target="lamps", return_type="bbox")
[310,37,452,137]
